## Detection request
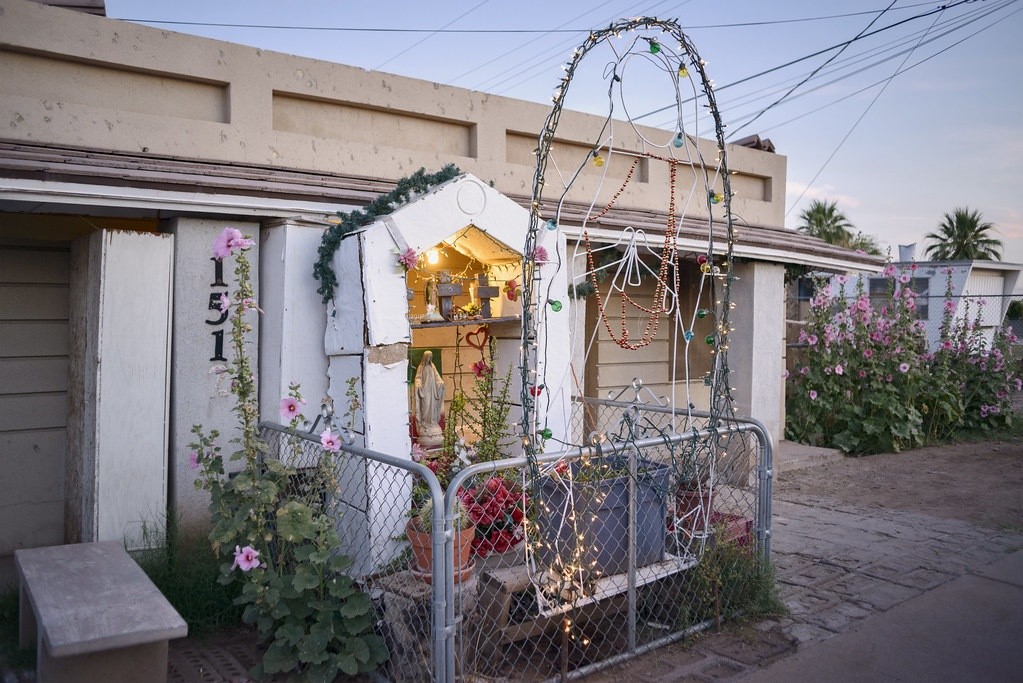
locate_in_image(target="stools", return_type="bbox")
[379,570,476,673]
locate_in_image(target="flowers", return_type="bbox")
[410,441,525,556]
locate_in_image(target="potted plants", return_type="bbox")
[532,457,673,579]
[678,468,718,529]
[406,492,478,585]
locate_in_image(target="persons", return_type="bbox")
[414,350,446,425]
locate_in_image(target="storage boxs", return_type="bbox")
[664,512,759,552]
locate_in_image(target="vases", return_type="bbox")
[474,537,529,575]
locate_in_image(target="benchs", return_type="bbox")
[474,565,640,660]
[14,541,188,683]
